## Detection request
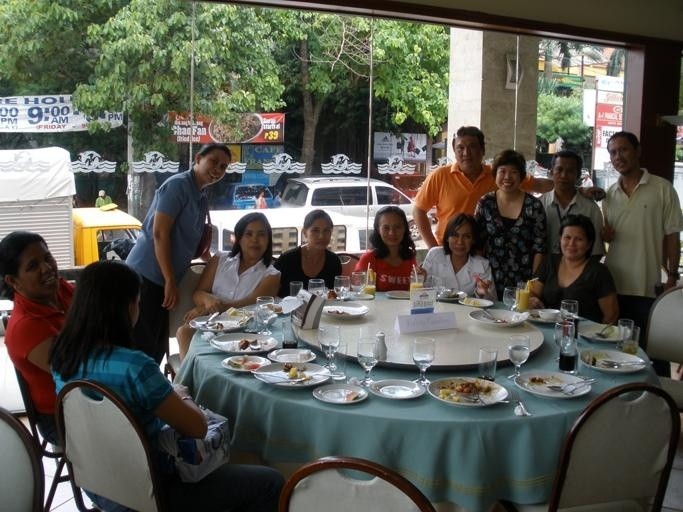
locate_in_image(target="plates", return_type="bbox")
[311,383,369,406]
[268,349,317,364]
[428,375,508,407]
[348,294,375,299]
[320,298,370,319]
[210,332,278,356]
[514,369,593,399]
[189,315,244,332]
[580,347,646,377]
[367,378,427,401]
[466,308,530,328]
[523,305,579,323]
[254,362,333,390]
[383,289,410,299]
[430,288,467,302]
[220,355,270,374]
[457,296,494,307]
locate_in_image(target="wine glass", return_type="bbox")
[351,271,366,297]
[255,295,273,337]
[558,299,579,321]
[318,324,341,373]
[507,334,531,382]
[305,278,325,291]
[412,336,435,388]
[553,321,577,363]
[356,336,379,387]
[332,274,350,299]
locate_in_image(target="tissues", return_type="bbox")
[282,288,327,329]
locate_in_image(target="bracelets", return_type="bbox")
[182,396,193,400]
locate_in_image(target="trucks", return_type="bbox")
[0,149,142,261]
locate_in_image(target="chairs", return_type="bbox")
[279,456,504,512]
[646,286,683,412]
[0,407,44,512]
[3,316,64,512]
[336,253,360,277]
[56,381,169,512]
[165,263,208,382]
[510,383,681,512]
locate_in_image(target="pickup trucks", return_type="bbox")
[206,177,421,240]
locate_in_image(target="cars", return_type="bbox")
[217,182,276,208]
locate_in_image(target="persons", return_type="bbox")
[48,260,285,511]
[95,190,112,208]
[125,143,232,366]
[273,210,343,299]
[412,127,683,326]
[177,212,282,364]
[473,149,547,301]
[257,190,281,209]
[413,127,606,251]
[536,151,606,264]
[526,215,620,325]
[416,212,497,301]
[0,229,75,447]
[601,131,683,325]
[353,206,417,292]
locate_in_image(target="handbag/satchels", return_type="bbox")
[194,224,212,258]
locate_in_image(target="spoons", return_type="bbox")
[548,378,598,396]
[602,356,654,371]
[594,320,618,339]
[580,321,631,344]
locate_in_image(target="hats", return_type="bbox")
[99,190,105,197]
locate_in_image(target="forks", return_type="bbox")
[510,389,533,418]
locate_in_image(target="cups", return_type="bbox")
[363,270,378,292]
[622,327,641,354]
[327,340,348,383]
[476,346,497,381]
[539,307,561,322]
[408,274,426,288]
[242,305,257,334]
[290,281,303,298]
[559,336,576,377]
[615,316,634,348]
[279,317,301,349]
